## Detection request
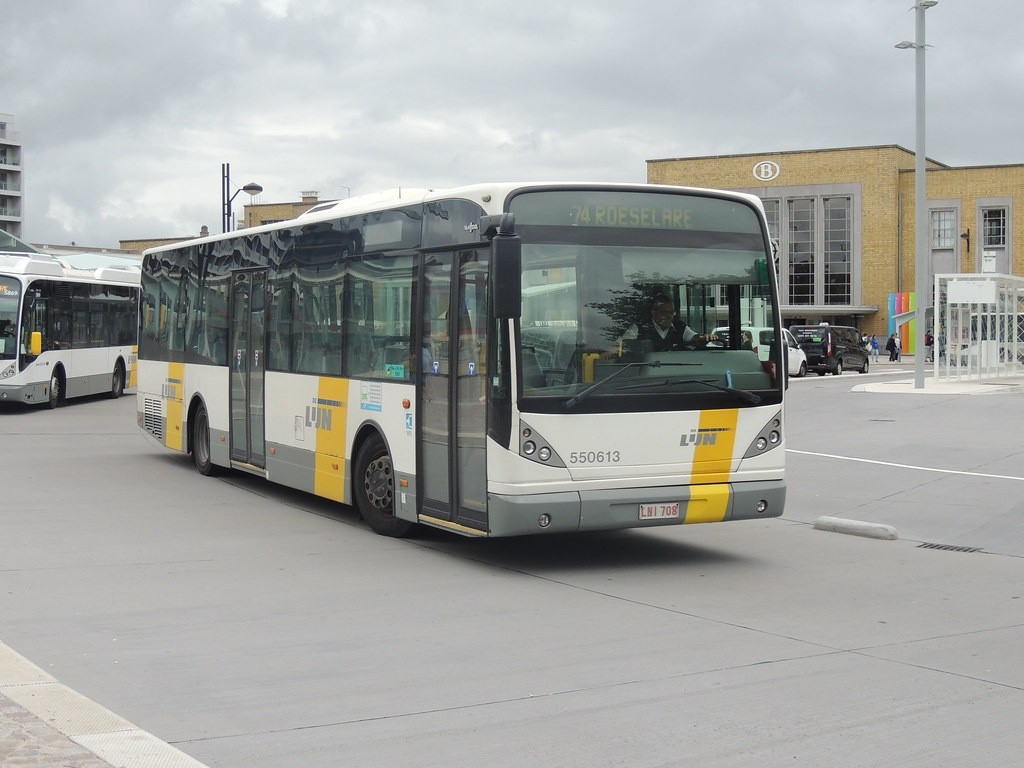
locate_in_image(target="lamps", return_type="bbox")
[960,229,970,240]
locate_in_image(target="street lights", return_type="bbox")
[222,163,263,233]
[894,0,939,388]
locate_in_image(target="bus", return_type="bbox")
[0,250,138,410]
[134,181,786,538]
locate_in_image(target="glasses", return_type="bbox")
[659,309,676,316]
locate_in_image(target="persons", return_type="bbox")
[5,315,17,337]
[862,333,880,363]
[926,331,947,362]
[598,295,719,361]
[742,331,753,350]
[885,332,902,363]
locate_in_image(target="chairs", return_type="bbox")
[520,331,585,389]
[321,354,341,376]
[197,332,227,365]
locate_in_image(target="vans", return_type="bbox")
[789,322,869,375]
[705,326,808,379]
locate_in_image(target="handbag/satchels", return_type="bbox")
[865,343,872,352]
